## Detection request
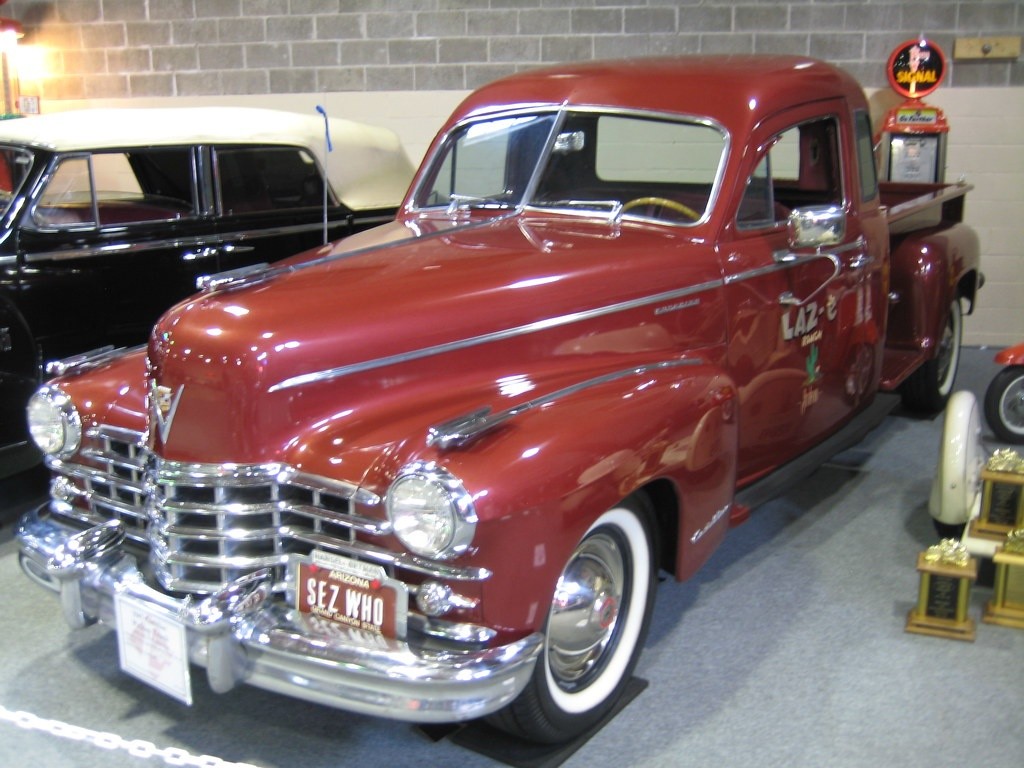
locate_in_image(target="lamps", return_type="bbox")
[0,16,25,55]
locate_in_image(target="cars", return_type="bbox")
[0,106,417,480]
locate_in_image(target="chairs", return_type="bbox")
[99,205,187,224]
[36,206,92,224]
[551,189,657,218]
[658,193,791,224]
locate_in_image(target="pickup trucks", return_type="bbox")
[16,54,984,743]
